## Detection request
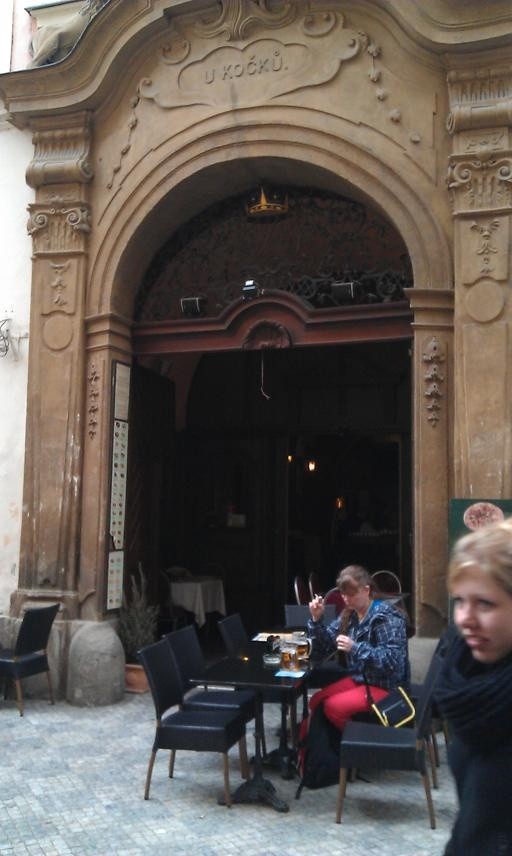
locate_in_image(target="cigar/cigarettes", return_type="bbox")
[314,593,319,597]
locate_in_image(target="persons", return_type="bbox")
[298,564,412,788]
[419,518,512,856]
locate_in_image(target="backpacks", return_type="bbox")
[299,693,342,790]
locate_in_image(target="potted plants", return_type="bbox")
[118,601,160,693]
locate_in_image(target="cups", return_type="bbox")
[265,632,312,673]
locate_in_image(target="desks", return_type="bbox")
[165,574,226,646]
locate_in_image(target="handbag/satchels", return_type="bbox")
[371,684,414,731]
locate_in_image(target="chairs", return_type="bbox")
[0,602,62,717]
[284,569,412,633]
